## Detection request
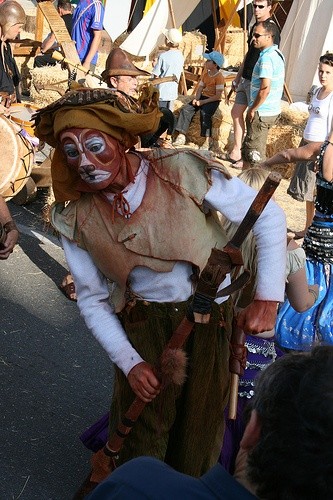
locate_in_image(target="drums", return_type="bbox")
[0,113,34,202]
[3,102,46,152]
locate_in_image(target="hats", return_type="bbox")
[100,48,152,80]
[202,51,224,68]
[162,29,183,44]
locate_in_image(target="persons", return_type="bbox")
[221,166,319,452]
[84,344,333,500]
[217,0,280,171]
[58,48,151,301]
[262,127,333,348]
[172,51,225,157]
[71,0,105,74]
[286,53,333,240]
[33,0,74,69]
[0,194,19,260]
[0,0,26,103]
[151,28,185,110]
[31,87,288,500]
[240,20,285,172]
[152,55,160,67]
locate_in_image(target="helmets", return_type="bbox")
[0,1,26,37]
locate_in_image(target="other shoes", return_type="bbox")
[232,159,244,168]
[172,136,189,146]
[73,465,113,500]
[199,138,214,150]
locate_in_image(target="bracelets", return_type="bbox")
[1,221,18,237]
[309,289,318,304]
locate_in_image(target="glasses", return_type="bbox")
[252,4,265,9]
[252,33,262,38]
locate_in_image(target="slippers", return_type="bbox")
[59,271,78,302]
[217,152,243,163]
